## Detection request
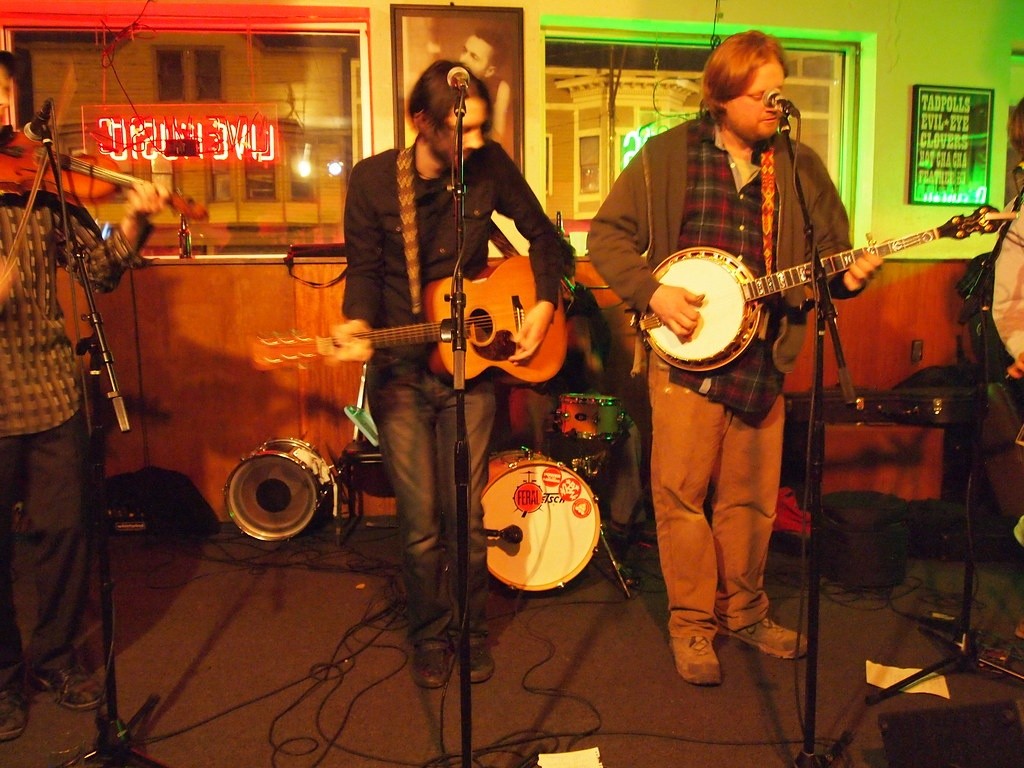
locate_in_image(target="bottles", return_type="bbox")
[102,222,110,240]
[555,211,564,241]
[178,213,191,259]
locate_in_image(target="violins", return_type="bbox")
[0,124,209,221]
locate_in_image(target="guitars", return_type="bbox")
[245,256,565,385]
[623,205,1024,374]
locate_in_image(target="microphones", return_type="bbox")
[24,99,52,142]
[763,88,800,118]
[447,67,470,90]
[499,526,523,544]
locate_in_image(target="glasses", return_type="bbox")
[739,85,784,102]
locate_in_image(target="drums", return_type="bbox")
[482,450,601,591]
[224,438,333,542]
[554,393,621,440]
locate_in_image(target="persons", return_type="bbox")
[0,50,168,739]
[991,96,1024,638]
[585,30,882,685]
[460,29,514,161]
[331,58,576,687]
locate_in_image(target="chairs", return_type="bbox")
[333,357,394,547]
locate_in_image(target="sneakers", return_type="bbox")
[716,615,807,658]
[409,643,450,689]
[671,636,722,687]
[32,651,107,712]
[454,638,494,683]
[0,665,27,741]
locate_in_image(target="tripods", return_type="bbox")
[40,131,174,768]
[864,184,1024,707]
